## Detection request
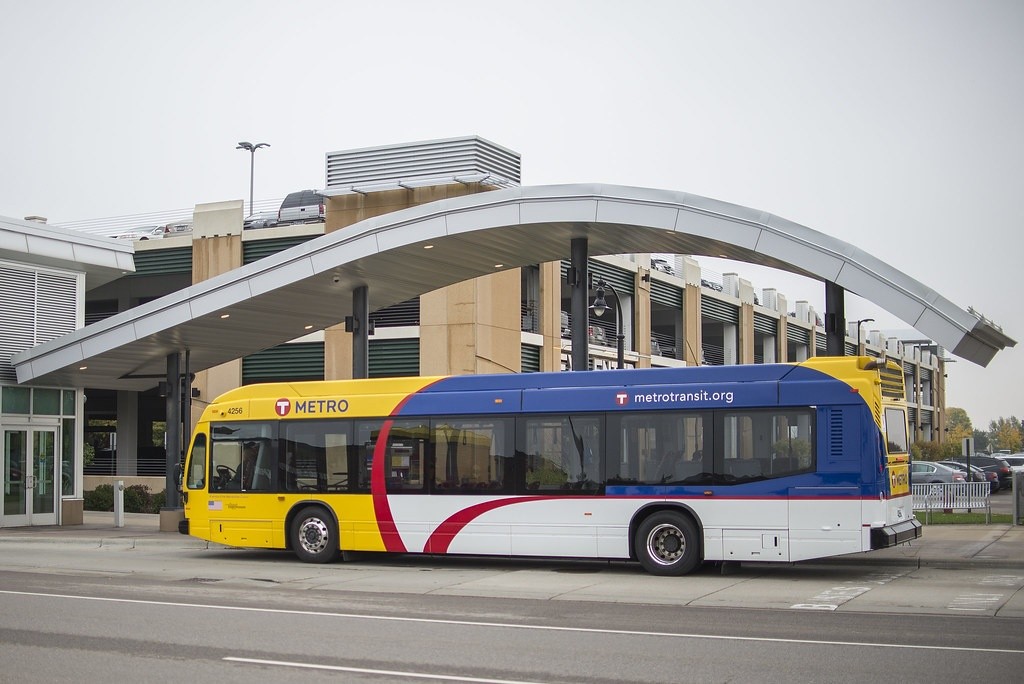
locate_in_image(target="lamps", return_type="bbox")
[567,267,576,286]
[919,426,948,432]
[642,274,650,282]
[588,273,593,289]
[159,381,171,398]
[369,319,375,335]
[346,316,358,332]
[825,313,835,332]
[944,374,947,378]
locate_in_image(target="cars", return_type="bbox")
[910,460,967,498]
[984,471,999,492]
[521,256,824,356]
[114,211,278,241]
[937,462,986,491]
[10,459,73,496]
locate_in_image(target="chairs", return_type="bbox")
[651,449,796,484]
[240,443,261,490]
[367,476,541,490]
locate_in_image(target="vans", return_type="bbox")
[994,456,1024,472]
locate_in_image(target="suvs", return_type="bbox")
[946,457,1012,489]
[278,189,325,224]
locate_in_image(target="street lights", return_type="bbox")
[236,141,271,215]
[589,278,624,369]
[857,318,875,357]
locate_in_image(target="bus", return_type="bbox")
[174,356,923,578]
